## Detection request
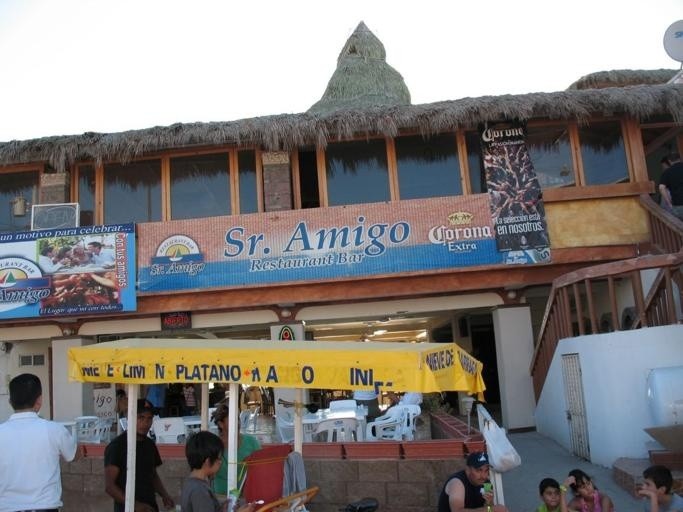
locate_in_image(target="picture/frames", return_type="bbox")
[31,203,80,231]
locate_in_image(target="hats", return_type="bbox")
[211,404,228,423]
[467,452,489,468]
[136,398,161,415]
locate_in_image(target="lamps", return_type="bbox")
[281,310,292,318]
[62,328,76,336]
[507,290,519,298]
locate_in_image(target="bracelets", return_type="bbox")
[486,504,492,512]
[559,484,568,492]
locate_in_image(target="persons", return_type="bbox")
[400,392,422,409]
[366,393,399,437]
[352,391,377,434]
[658,150,682,221]
[164,383,225,418]
[103,396,176,512]
[115,389,127,419]
[0,371,80,512]
[208,404,227,448]
[631,464,682,512]
[659,153,671,214]
[535,478,562,512]
[436,451,508,512]
[557,469,615,512]
[38,238,120,308]
[179,429,257,512]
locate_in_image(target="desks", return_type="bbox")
[74,416,98,443]
[185,415,201,433]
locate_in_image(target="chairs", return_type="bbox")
[155,418,186,444]
[89,417,114,444]
[275,392,423,441]
[250,406,259,432]
[239,409,249,432]
[120,418,128,431]
